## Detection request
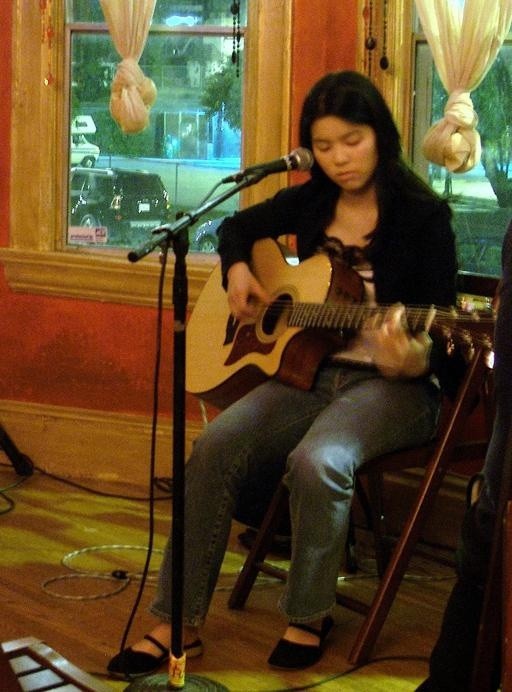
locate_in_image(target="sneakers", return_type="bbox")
[267,613,333,672]
[107,633,204,680]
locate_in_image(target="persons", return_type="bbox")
[106,69,460,679]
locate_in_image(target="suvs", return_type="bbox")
[69,167,126,246]
[108,168,173,244]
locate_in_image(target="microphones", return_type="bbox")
[222,146,315,183]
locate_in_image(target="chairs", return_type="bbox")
[229,312,490,665]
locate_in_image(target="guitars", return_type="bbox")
[185,236,506,410]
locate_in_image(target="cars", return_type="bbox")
[71,133,100,168]
[194,214,236,253]
